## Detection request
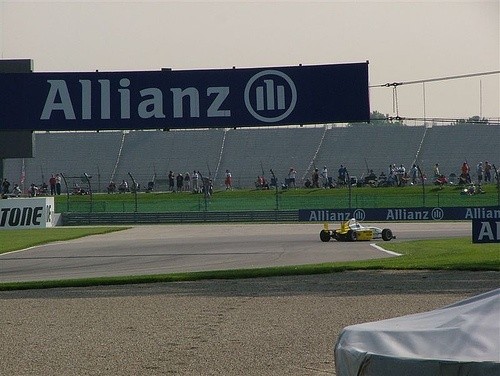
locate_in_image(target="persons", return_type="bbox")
[367,162,500,196]
[255,162,350,190]
[109,166,235,195]
[2,172,89,199]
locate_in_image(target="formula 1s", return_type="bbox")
[319,222,393,242]
